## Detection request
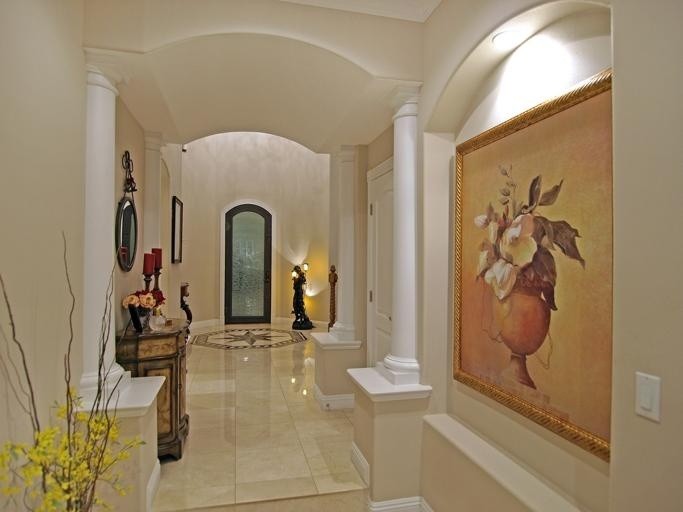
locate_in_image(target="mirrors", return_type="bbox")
[116,150,138,272]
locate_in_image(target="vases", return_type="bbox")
[490,285,551,390]
[130,317,146,331]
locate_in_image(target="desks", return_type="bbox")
[115,316,190,461]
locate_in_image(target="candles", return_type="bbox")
[143,252,155,274]
[153,249,161,268]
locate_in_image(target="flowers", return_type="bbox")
[122,290,166,317]
[472,159,586,310]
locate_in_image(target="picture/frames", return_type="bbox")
[452,66,613,462]
[172,195,183,264]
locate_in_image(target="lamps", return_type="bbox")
[303,263,308,270]
